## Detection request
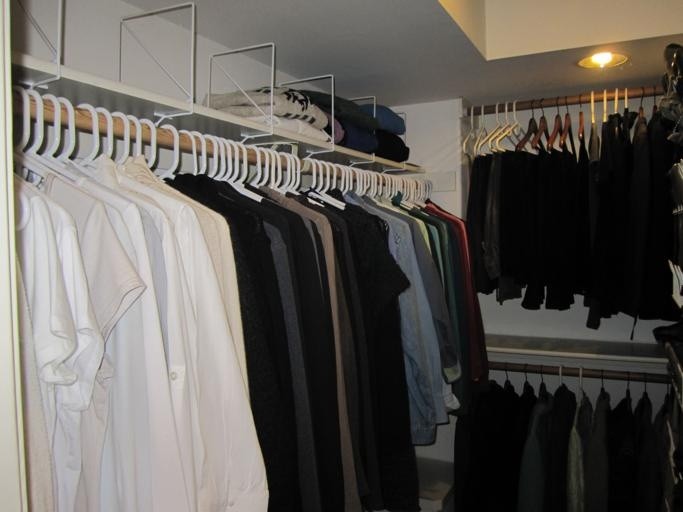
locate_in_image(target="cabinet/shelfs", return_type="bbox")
[462,84,683,507]
[8,2,435,508]
